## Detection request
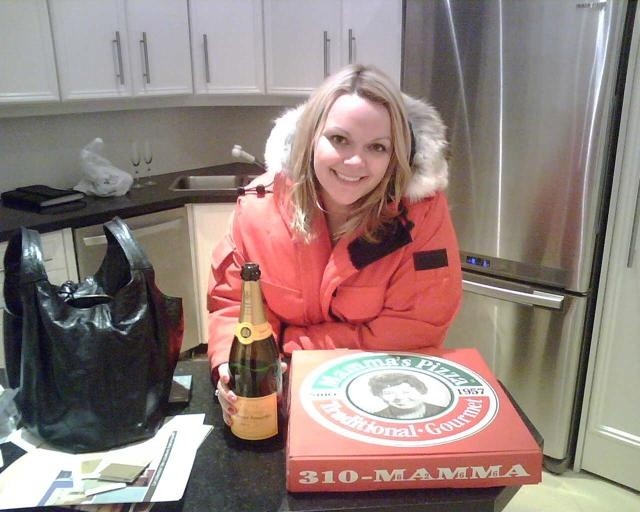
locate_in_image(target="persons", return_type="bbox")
[368,371,448,421]
[204,61,464,429]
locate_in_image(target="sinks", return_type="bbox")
[168,173,264,192]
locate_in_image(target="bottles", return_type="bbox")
[227,264,283,449]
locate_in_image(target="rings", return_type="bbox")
[214,391,223,398]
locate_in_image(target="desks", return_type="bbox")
[1,362,545,512]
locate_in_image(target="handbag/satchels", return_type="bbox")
[2,216,184,452]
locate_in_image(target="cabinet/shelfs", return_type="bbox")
[262,0,403,108]
[0,0,59,119]
[188,0,266,109]
[47,0,194,115]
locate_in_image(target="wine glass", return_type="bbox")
[130,140,144,188]
[143,141,158,186]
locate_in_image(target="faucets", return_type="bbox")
[227,142,265,170]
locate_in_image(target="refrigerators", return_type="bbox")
[399,3,633,473]
